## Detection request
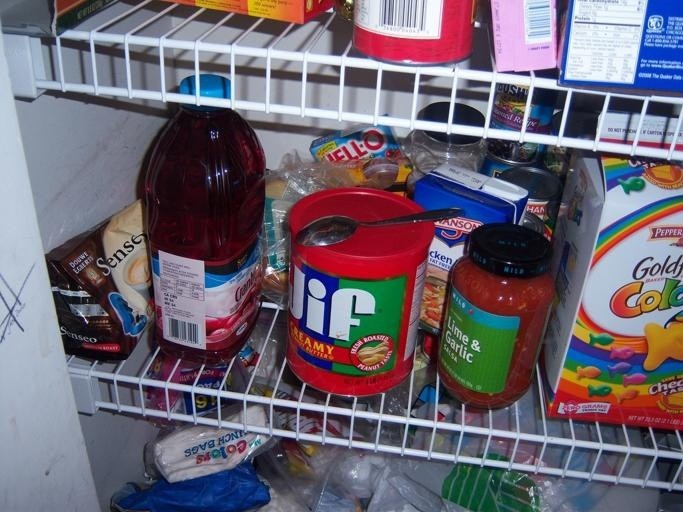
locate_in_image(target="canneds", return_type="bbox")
[284,188,434,396]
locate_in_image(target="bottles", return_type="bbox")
[351,0,475,67]
[403,102,485,192]
[498,168,559,224]
[487,69,559,163]
[485,153,519,179]
[438,224,554,410]
[141,74,265,364]
[519,209,553,245]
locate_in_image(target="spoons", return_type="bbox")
[294,205,471,247]
